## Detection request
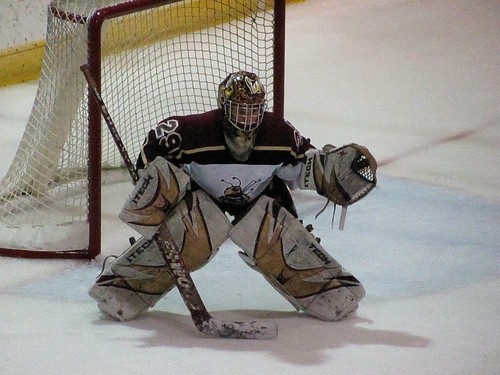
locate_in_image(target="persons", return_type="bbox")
[89,71,377,321]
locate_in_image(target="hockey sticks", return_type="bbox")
[80,63,279,341]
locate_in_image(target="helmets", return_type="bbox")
[217,71,265,138]
